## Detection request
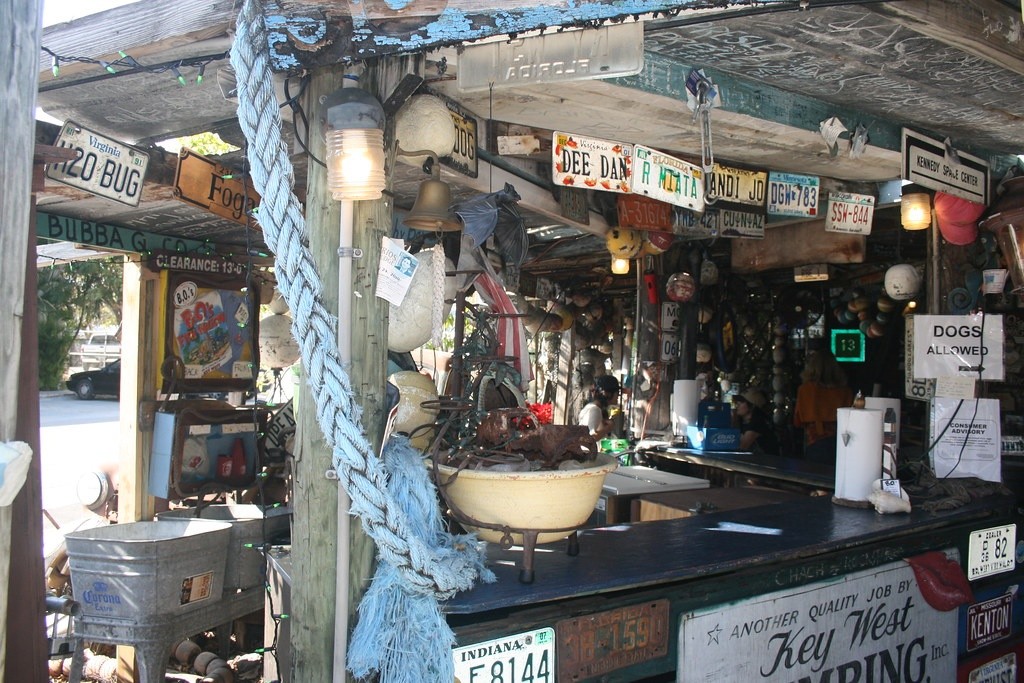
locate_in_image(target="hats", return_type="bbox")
[731,387,767,410]
[934,189,985,245]
[595,375,622,392]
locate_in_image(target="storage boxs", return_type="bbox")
[64,520,233,621]
[155,504,290,592]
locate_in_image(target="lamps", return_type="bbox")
[900,183,932,231]
[324,101,386,202]
[612,254,629,274]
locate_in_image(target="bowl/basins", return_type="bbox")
[419,452,619,543]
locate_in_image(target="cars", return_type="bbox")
[66,357,121,399]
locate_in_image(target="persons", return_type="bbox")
[736,388,768,450]
[792,351,854,453]
[578,375,618,454]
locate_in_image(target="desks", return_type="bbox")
[600,465,708,525]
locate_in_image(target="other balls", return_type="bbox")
[771,325,786,427]
[512,266,616,351]
[605,225,674,260]
[830,294,893,340]
